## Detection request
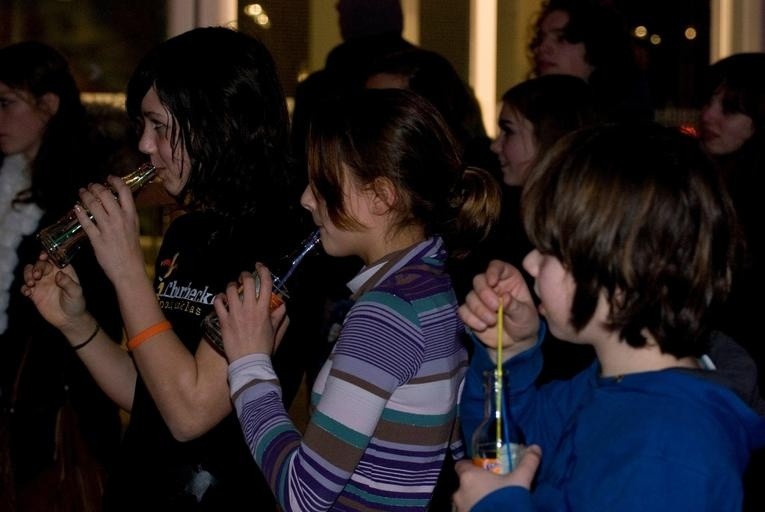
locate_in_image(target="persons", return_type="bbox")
[450,113,765,512]
[489,73,607,190]
[17,25,330,511]
[0,38,125,512]
[289,0,496,409]
[524,1,653,121]
[692,51,765,403]
[342,40,426,94]
[213,86,509,511]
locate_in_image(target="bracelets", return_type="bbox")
[69,321,101,353]
[119,319,173,355]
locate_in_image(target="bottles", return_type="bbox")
[470,367,529,477]
[195,226,322,356]
[36,160,162,269]
